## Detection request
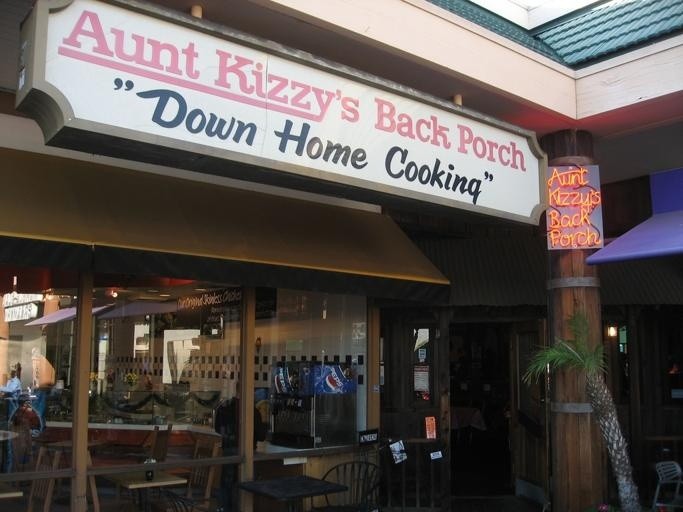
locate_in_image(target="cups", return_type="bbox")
[315,365,350,393]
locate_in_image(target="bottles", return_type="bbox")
[9,397,41,473]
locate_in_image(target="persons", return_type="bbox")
[0,371,22,408]
[214,381,265,512]
[9,394,41,465]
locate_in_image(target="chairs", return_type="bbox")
[321,461,385,511]
[11,419,74,512]
[166,439,227,512]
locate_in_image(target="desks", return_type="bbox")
[239,471,349,511]
[91,459,188,511]
